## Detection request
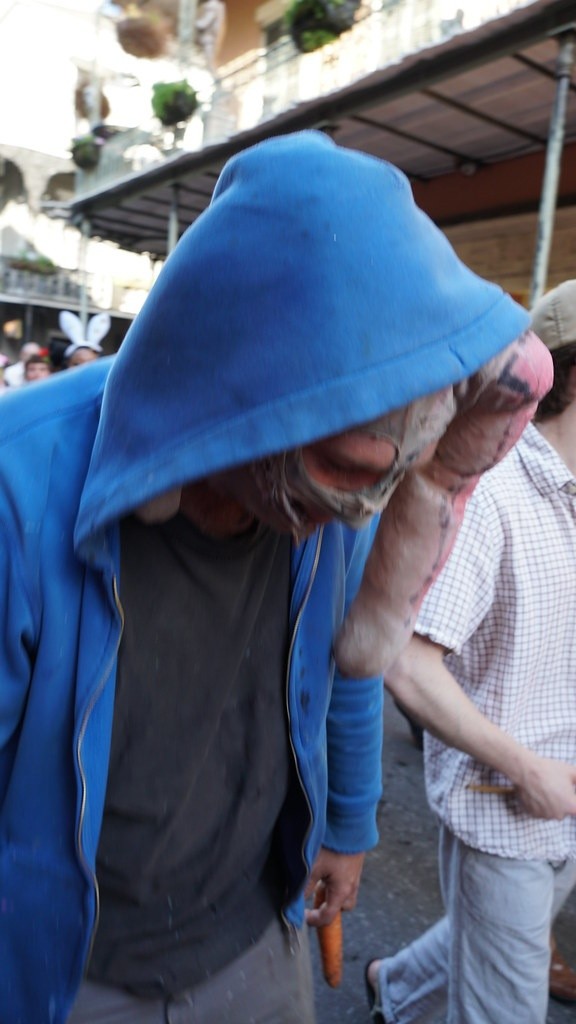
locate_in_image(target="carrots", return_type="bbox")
[313,881,341,986]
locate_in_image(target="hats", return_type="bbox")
[528,279,576,351]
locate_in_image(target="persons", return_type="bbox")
[1,130,576,1024]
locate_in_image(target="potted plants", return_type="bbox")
[71,134,103,168]
[284,0,362,53]
[151,79,199,124]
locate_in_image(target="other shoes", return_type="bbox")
[363,958,396,1024]
[549,947,576,1006]
